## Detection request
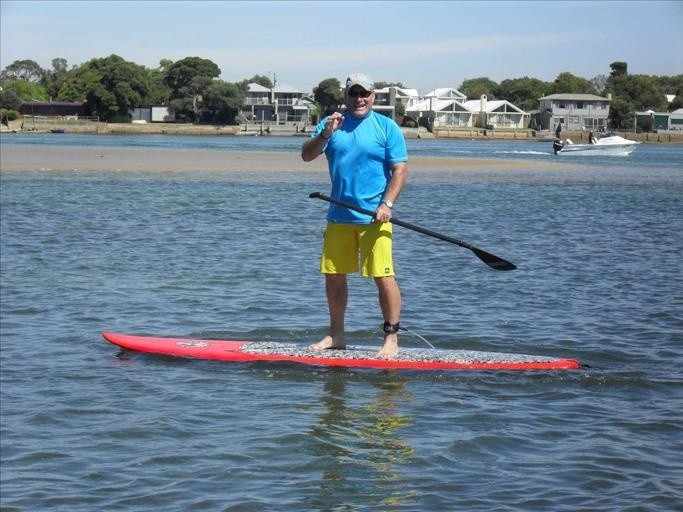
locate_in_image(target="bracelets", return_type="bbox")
[320,129,330,140]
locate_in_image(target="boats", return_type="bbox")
[557,136,640,156]
[51,129,65,133]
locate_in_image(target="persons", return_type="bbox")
[588,130,597,145]
[301,73,407,360]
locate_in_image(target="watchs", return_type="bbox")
[381,198,393,209]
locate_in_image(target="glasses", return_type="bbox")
[348,90,373,97]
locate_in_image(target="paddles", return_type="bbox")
[309,192,518,270]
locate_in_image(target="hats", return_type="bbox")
[345,73,375,92]
